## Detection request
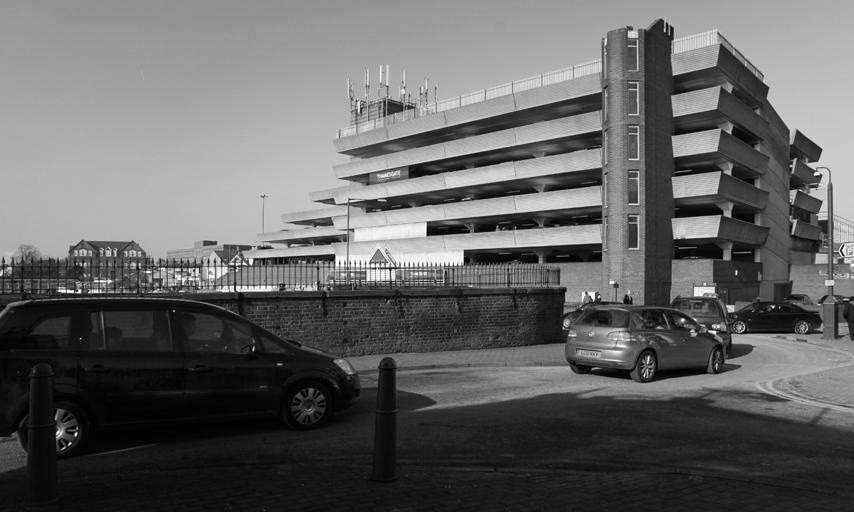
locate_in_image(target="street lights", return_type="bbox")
[814,166,834,296]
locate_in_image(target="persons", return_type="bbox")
[581,291,592,305]
[843,296,853,340]
[183,311,199,353]
[622,289,632,304]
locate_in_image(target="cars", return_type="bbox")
[564,302,729,384]
[670,293,732,355]
[729,301,822,334]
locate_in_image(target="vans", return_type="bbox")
[0,297,363,461]
